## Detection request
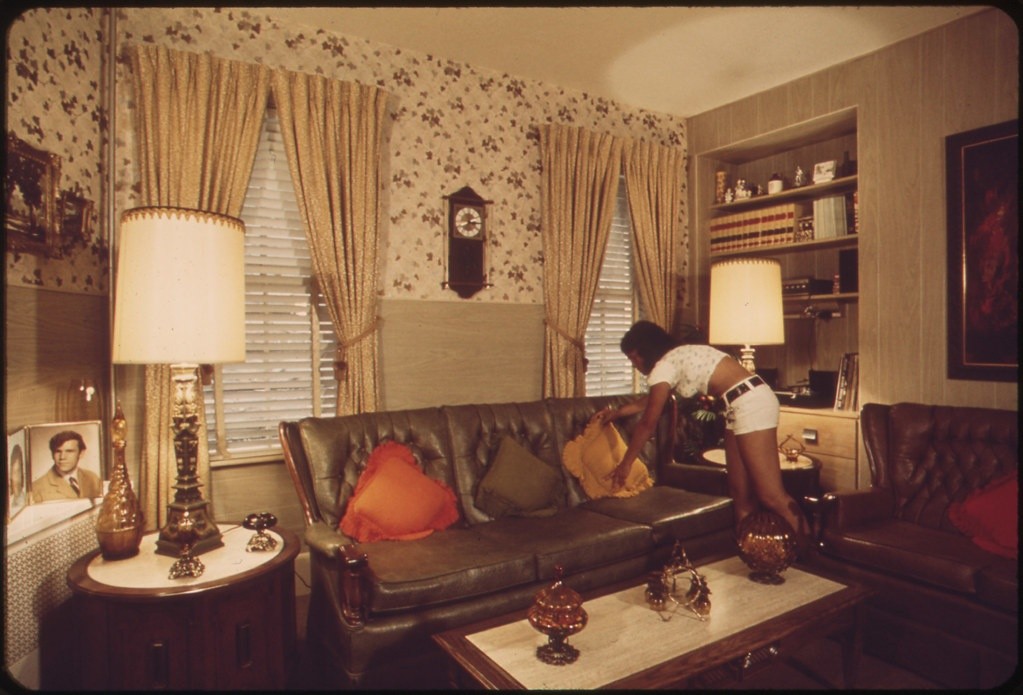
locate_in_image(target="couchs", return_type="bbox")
[276,393,737,695]
[799,402,1022,695]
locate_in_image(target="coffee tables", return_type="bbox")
[431,547,871,695]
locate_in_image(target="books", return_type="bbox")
[832,250,840,295]
[833,352,858,413]
[709,191,859,255]
[782,275,815,297]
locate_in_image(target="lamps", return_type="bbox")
[706,255,785,375]
[115,204,243,553]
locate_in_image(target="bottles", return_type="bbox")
[767,174,783,195]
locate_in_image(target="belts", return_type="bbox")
[719,375,765,407]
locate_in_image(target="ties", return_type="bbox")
[69,478,79,496]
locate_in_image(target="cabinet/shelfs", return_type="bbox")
[694,105,866,496]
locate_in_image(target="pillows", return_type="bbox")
[473,430,566,525]
[560,410,656,498]
[949,472,1019,557]
[337,439,459,544]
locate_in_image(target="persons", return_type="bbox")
[8,430,103,519]
[595,320,811,546]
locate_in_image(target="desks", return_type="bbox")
[65,520,300,694]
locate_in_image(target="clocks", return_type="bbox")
[453,203,484,240]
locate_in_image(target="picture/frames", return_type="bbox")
[945,120,1021,384]
[24,418,106,505]
[5,425,27,520]
